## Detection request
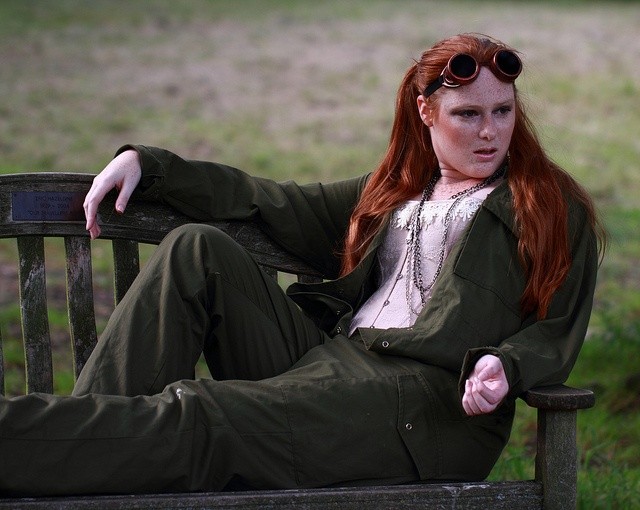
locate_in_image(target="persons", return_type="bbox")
[1,34,607,496]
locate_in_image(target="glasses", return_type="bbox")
[438,47,522,89]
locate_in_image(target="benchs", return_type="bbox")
[1,175,597,504]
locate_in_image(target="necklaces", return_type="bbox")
[406,173,502,316]
